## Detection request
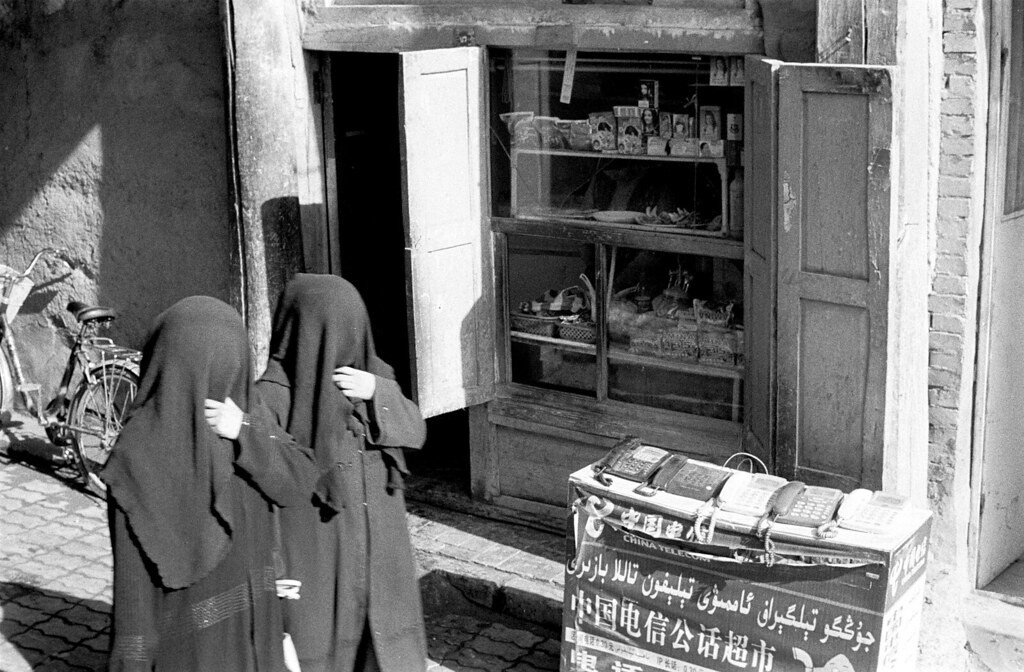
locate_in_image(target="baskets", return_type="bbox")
[558,324,596,344]
[510,311,559,337]
[0,264,34,324]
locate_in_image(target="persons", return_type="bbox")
[593,56,744,156]
[255,273,429,672]
[99,295,321,672]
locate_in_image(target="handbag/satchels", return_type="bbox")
[579,274,640,337]
[275,580,301,672]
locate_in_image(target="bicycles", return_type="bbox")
[0,247,143,500]
